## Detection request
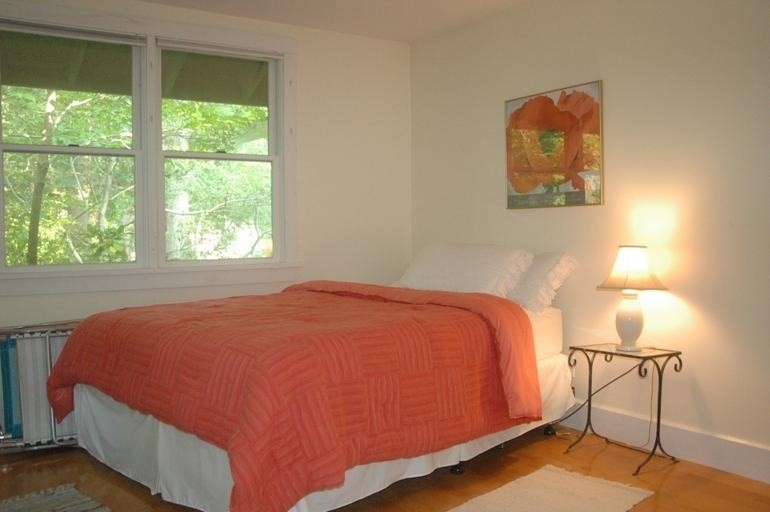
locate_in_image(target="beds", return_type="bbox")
[46,280,574,512]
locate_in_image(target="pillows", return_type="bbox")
[506,249,578,312]
[395,239,532,298]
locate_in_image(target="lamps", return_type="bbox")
[596,245,670,352]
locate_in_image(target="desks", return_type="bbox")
[565,342,683,477]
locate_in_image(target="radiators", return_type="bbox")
[1,330,77,451]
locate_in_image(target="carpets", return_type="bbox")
[449,465,654,512]
[0,483,110,512]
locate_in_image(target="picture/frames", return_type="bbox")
[504,80,604,210]
